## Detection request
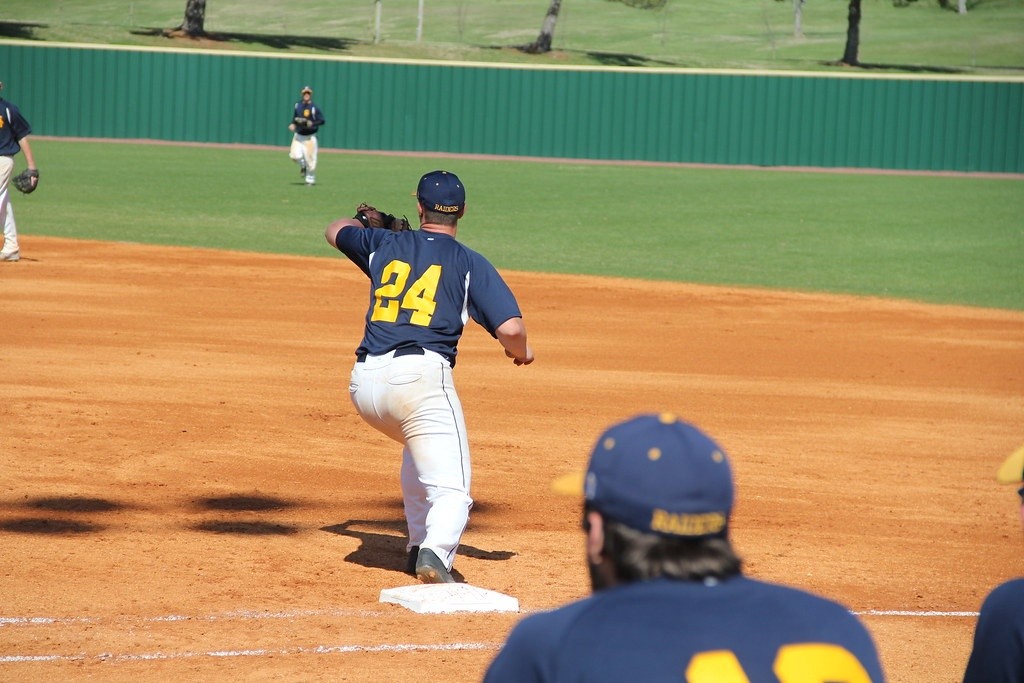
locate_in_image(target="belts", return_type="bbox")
[356,347,455,368]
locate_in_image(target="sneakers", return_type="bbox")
[416,548,455,583]
[404,546,419,574]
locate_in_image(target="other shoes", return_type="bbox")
[0,250,20,262]
[301,166,305,176]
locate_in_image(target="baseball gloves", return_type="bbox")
[13,169,39,193]
[356,202,413,230]
[294,117,309,129]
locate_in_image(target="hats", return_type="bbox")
[997,447,1024,483]
[411,170,465,214]
[552,412,733,540]
[302,86,312,94]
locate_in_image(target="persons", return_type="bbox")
[324,169,534,584]
[961,445,1024,683]
[0,81,39,262]
[482,411,887,683]
[287,84,325,185]
